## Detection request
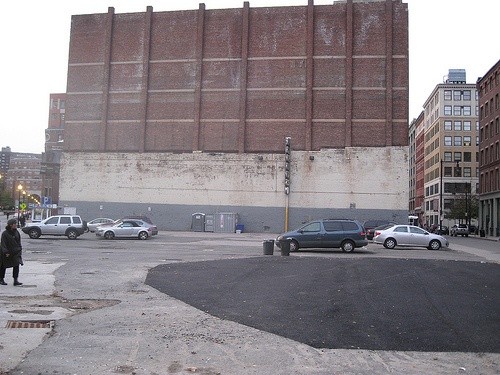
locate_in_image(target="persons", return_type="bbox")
[0,219,24,286]
[6,209,31,227]
[417,220,432,233]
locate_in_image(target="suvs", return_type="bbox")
[450,223,469,237]
[275,218,368,254]
[21,215,87,240]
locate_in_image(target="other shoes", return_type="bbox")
[14,283,23,287]
[0,280,7,285]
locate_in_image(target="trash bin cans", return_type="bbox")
[480,230,485,237]
[279,240,290,256]
[263,239,274,255]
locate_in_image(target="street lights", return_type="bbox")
[21,190,40,220]
[17,184,22,228]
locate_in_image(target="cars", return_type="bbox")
[98,220,152,239]
[95,215,159,236]
[366,225,392,240]
[373,224,449,250]
[428,224,449,235]
[86,217,114,232]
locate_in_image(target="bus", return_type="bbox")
[408,216,419,227]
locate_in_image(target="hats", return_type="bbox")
[7,219,18,225]
